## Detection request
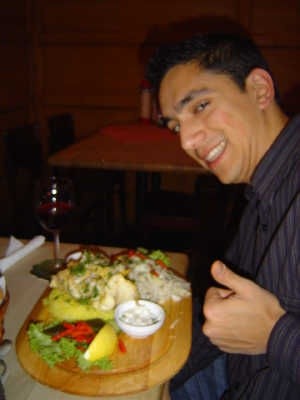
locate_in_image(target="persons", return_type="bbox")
[145,31,300,400]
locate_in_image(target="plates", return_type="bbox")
[15,264,194,397]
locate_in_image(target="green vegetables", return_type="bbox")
[54,247,146,307]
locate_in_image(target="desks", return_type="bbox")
[0,237,188,400]
[48,122,209,223]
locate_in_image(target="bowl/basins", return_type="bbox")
[112,297,167,338]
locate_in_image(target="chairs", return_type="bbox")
[5,117,246,251]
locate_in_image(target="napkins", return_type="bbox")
[0,235,45,272]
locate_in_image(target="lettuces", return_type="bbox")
[28,319,117,370]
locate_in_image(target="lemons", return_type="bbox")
[84,322,117,360]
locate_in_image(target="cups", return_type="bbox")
[0,290,10,346]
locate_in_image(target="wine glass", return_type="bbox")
[35,177,75,276]
